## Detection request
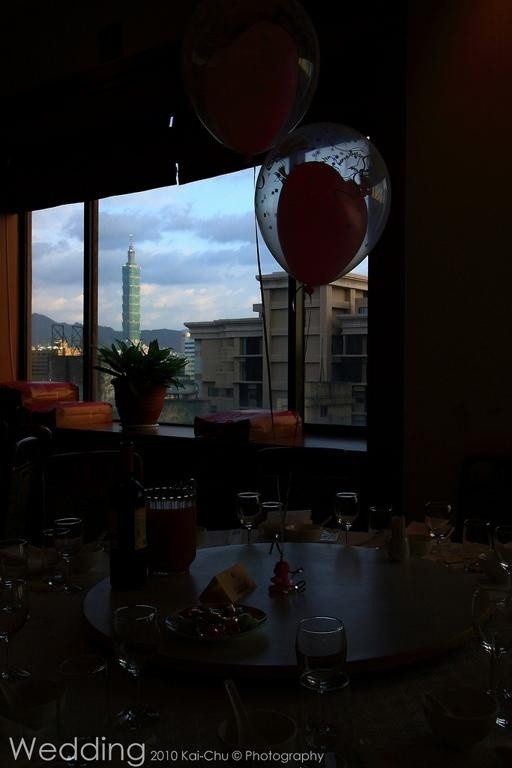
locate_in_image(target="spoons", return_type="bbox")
[223,677,269,749]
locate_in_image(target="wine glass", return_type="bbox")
[334,490,362,546]
[233,489,264,543]
[40,516,87,597]
[470,586,512,698]
[112,604,162,730]
[421,499,456,557]
[0,579,30,681]
[293,616,347,738]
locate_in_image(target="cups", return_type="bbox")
[368,503,394,546]
[261,500,287,529]
[143,484,200,579]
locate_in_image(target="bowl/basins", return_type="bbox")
[419,685,499,745]
[216,708,299,762]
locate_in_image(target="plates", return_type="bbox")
[164,601,269,646]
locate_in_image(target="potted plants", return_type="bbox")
[90,336,189,428]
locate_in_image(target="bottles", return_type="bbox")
[388,536,411,562]
[473,520,508,576]
[105,440,149,590]
